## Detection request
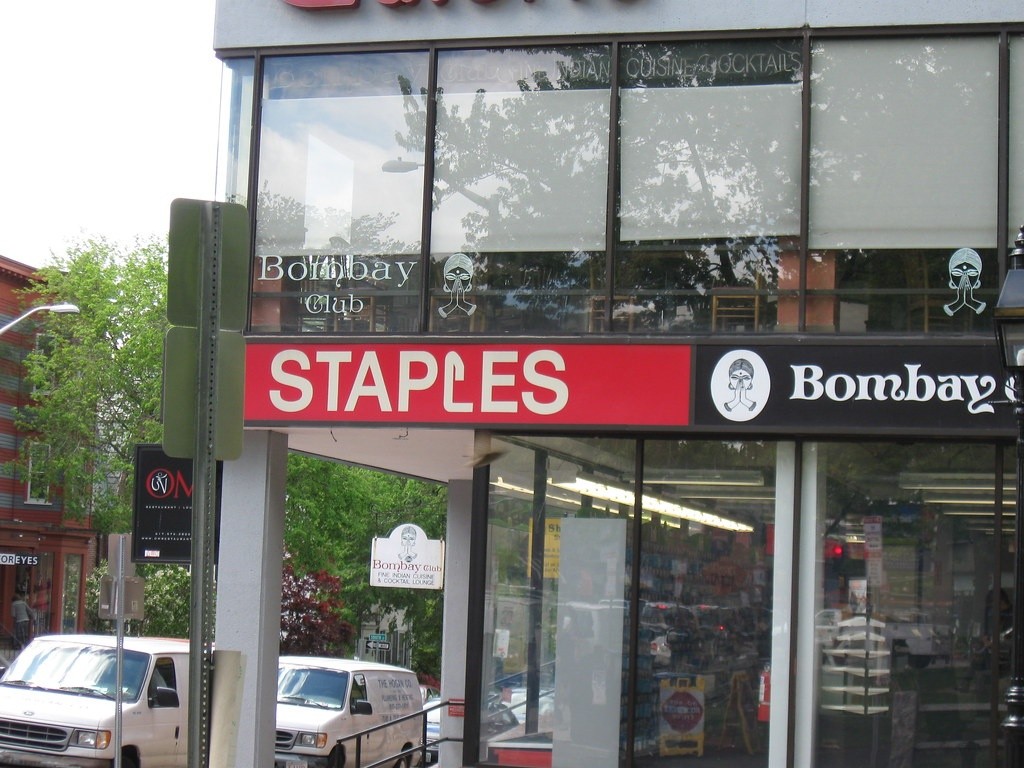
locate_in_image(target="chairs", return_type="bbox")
[902,248,976,332]
[429,257,488,335]
[586,257,638,332]
[298,255,347,333]
[709,261,766,333]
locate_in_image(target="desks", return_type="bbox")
[333,286,393,334]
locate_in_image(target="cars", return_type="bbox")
[500,687,554,724]
[419,684,440,708]
[599,603,855,675]
[419,697,519,765]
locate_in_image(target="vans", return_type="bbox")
[0,633,215,768]
[272,655,424,768]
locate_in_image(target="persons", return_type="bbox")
[11,593,35,648]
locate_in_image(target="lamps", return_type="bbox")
[547,469,759,535]
